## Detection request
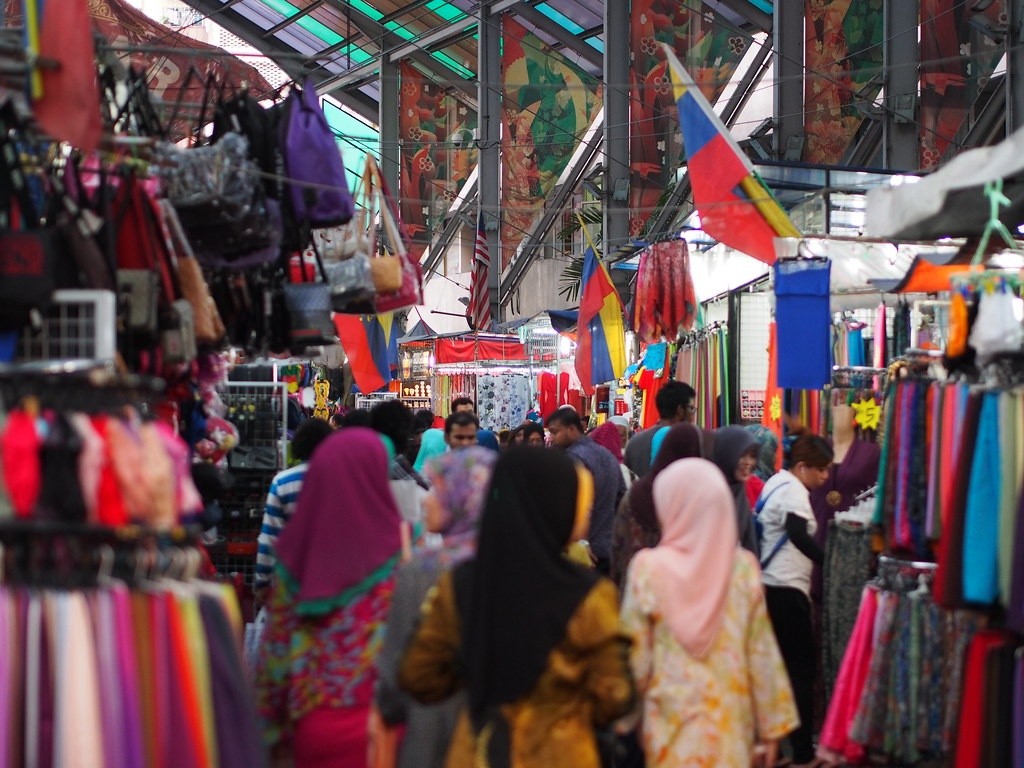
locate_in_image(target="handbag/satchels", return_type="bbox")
[0,39,426,361]
[239,607,266,699]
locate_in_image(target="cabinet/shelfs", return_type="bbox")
[396,338,435,411]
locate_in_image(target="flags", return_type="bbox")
[572,246,627,395]
[331,303,397,394]
[467,209,492,331]
[667,64,797,264]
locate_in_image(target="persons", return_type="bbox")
[825,404,881,509]
[258,380,834,768]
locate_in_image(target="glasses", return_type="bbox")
[683,405,697,415]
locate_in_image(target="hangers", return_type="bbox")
[677,318,726,352]
[1,514,237,606]
[863,551,940,606]
[824,308,1024,398]
